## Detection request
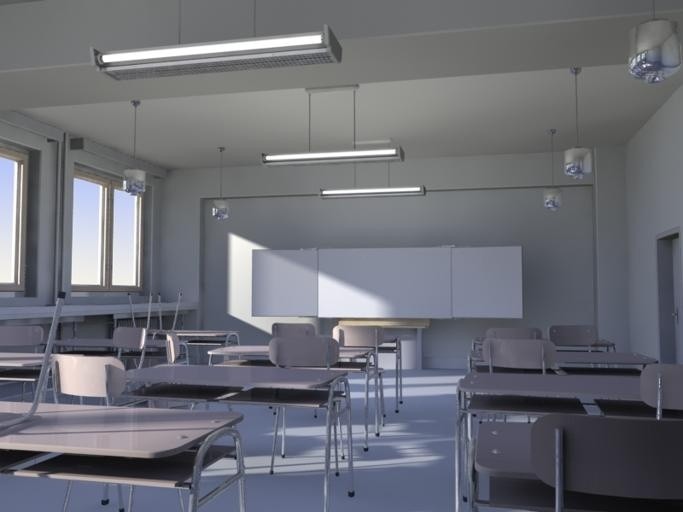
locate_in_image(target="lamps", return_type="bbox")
[122,101,148,196]
[212,146,232,220]
[564,66,593,179]
[542,127,563,212]
[316,139,428,198]
[627,0,683,86]
[260,82,403,164]
[89,0,342,81]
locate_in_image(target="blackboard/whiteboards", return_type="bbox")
[251,245,523,319]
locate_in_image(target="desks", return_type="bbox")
[448,373,675,512]
[470,348,659,371]
[471,336,616,351]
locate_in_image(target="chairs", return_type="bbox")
[485,326,541,340]
[471,337,552,506]
[0,290,432,512]
[548,324,616,373]
[527,408,683,510]
